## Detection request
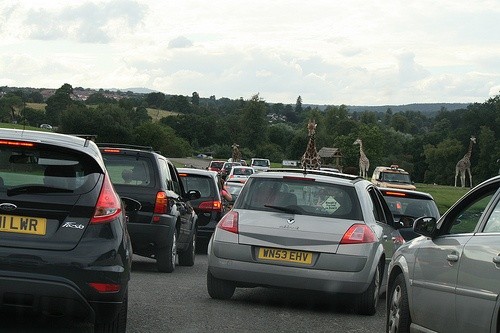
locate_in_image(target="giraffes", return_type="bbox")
[352,138,370,179]
[300,119,321,170]
[230,143,242,162]
[454,137,476,190]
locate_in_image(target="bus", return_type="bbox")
[371,164,417,192]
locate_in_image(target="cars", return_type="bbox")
[240,160,247,166]
[226,166,256,181]
[219,162,242,181]
[384,175,500,333]
[223,178,247,200]
[377,186,442,242]
[40,123,52,129]
[206,160,228,172]
[205,163,415,316]
[197,154,207,158]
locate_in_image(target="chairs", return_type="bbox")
[43,165,78,190]
[272,191,297,207]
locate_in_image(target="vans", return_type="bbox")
[247,158,271,174]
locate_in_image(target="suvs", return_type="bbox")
[0,128,143,333]
[175,167,227,255]
[95,141,202,274]
